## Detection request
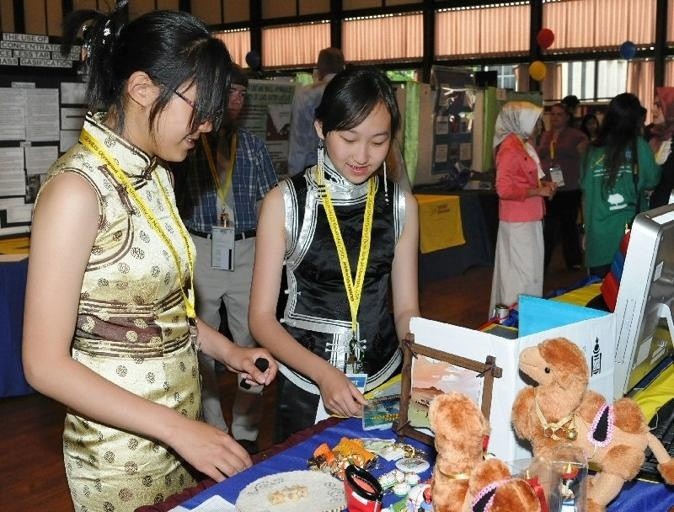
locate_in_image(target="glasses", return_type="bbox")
[156,78,225,125]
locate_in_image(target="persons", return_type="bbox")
[21,8,277,512]
[287,47,346,178]
[537,86,674,279]
[488,101,558,321]
[168,60,279,455]
[248,65,421,445]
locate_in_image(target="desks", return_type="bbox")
[0,236,35,399]
[412,174,497,291]
[133,277,674,512]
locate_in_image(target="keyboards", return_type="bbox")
[635,398,674,484]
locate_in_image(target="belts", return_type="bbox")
[188,226,256,241]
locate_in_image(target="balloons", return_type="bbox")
[537,28,554,49]
[528,61,546,81]
[621,41,636,59]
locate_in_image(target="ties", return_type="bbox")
[214,130,237,224]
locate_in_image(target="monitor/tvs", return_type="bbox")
[614,202,674,400]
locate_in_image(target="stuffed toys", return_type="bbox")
[512,337,674,512]
[429,391,541,512]
